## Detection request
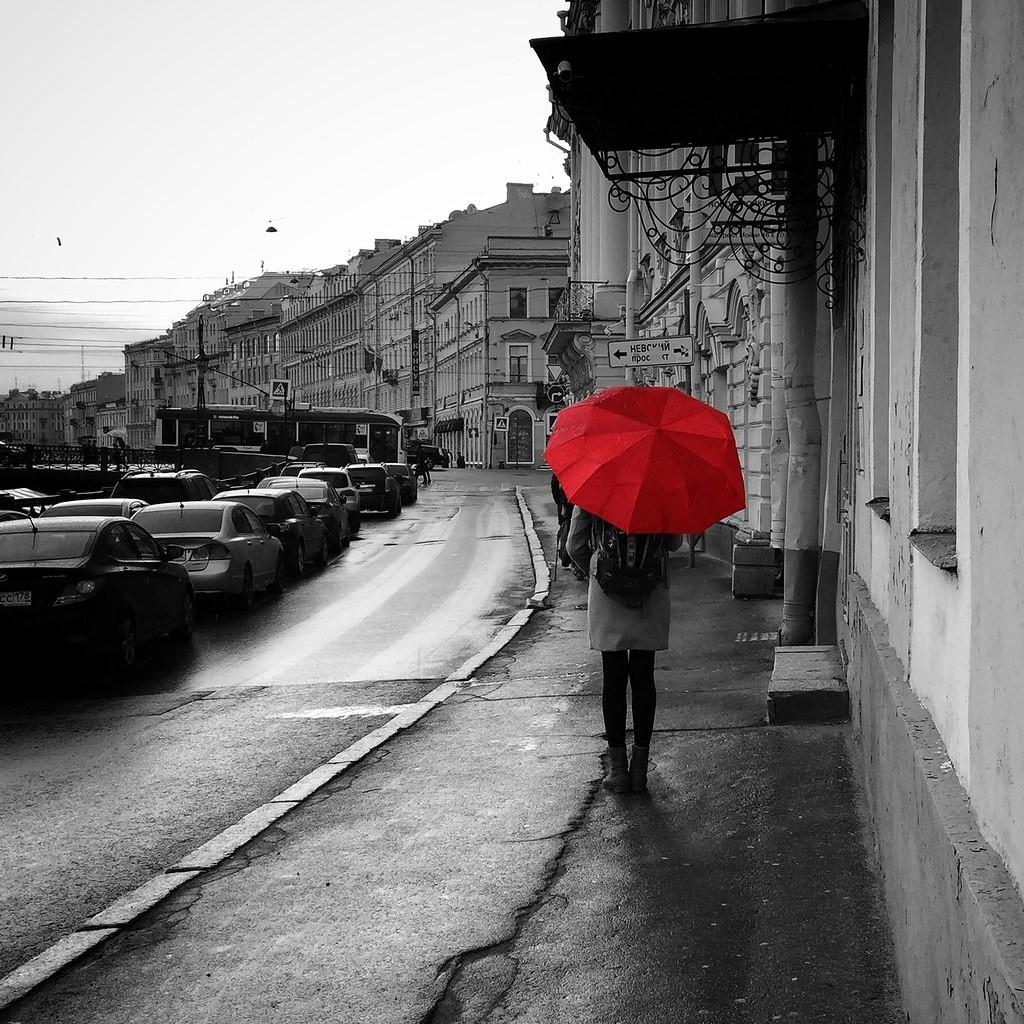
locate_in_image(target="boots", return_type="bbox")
[602,745,649,793]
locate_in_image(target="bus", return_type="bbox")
[152,402,414,476]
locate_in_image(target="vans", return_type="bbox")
[301,439,360,468]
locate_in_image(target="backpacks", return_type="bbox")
[593,515,669,609]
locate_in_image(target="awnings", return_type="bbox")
[434,417,464,433]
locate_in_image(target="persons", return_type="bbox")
[456,451,465,469]
[82,439,100,464]
[565,504,685,794]
[409,437,434,486]
[112,436,129,471]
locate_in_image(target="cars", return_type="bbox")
[267,478,351,556]
[130,499,288,613]
[0,515,196,696]
[212,488,332,578]
[39,497,150,520]
[379,462,418,505]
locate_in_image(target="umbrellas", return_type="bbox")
[107,429,129,438]
[542,385,746,533]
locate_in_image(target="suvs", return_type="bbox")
[298,465,361,534]
[109,468,220,507]
[278,460,327,480]
[342,462,403,517]
